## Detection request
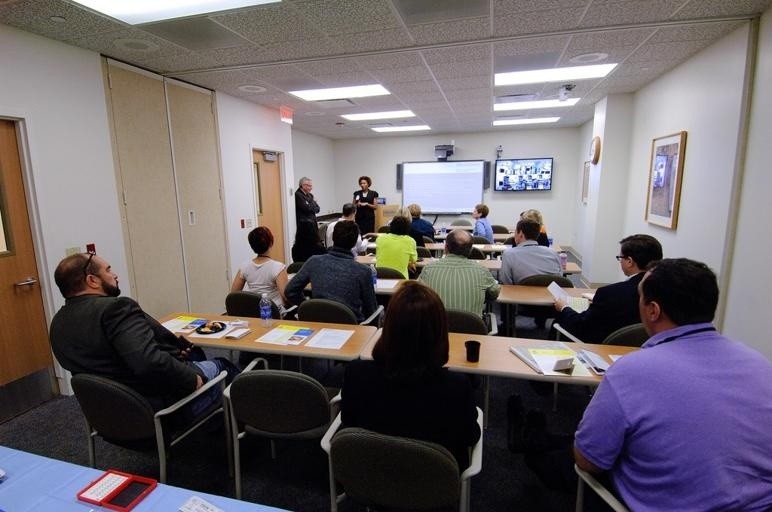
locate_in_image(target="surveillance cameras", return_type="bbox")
[497,144,504,157]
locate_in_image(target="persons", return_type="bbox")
[294,176,328,255]
[408,203,436,257]
[507,257,772,512]
[416,228,501,390]
[50,254,264,431]
[493,208,549,258]
[341,283,481,473]
[285,221,378,327]
[535,234,663,344]
[499,219,564,288]
[393,205,412,224]
[230,226,297,320]
[326,203,369,256]
[472,202,494,250]
[375,215,418,280]
[352,176,378,254]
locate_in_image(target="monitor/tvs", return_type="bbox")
[494,158,554,192]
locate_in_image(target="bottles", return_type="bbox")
[370,264,377,291]
[260,293,272,328]
[441,223,446,237]
[548,233,553,248]
[560,251,567,270]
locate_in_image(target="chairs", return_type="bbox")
[69,370,227,484]
[226,293,297,320]
[320,407,487,512]
[224,358,330,500]
[572,465,632,512]
[374,266,407,279]
[451,219,472,227]
[299,300,384,328]
[491,226,508,232]
[416,247,432,259]
[554,323,646,410]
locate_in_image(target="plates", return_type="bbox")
[195,323,227,336]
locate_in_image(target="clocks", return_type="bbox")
[589,137,600,163]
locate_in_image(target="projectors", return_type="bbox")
[557,84,577,102]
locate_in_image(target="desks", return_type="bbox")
[436,233,515,240]
[288,274,417,297]
[362,329,645,429]
[356,256,581,277]
[157,312,377,361]
[424,242,560,255]
[435,226,476,233]
[496,284,599,337]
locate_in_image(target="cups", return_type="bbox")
[465,340,481,363]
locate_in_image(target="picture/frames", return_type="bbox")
[645,131,687,230]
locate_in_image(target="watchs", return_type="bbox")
[362,237,367,241]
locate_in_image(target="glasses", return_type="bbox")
[84,250,96,278]
[615,255,625,262]
